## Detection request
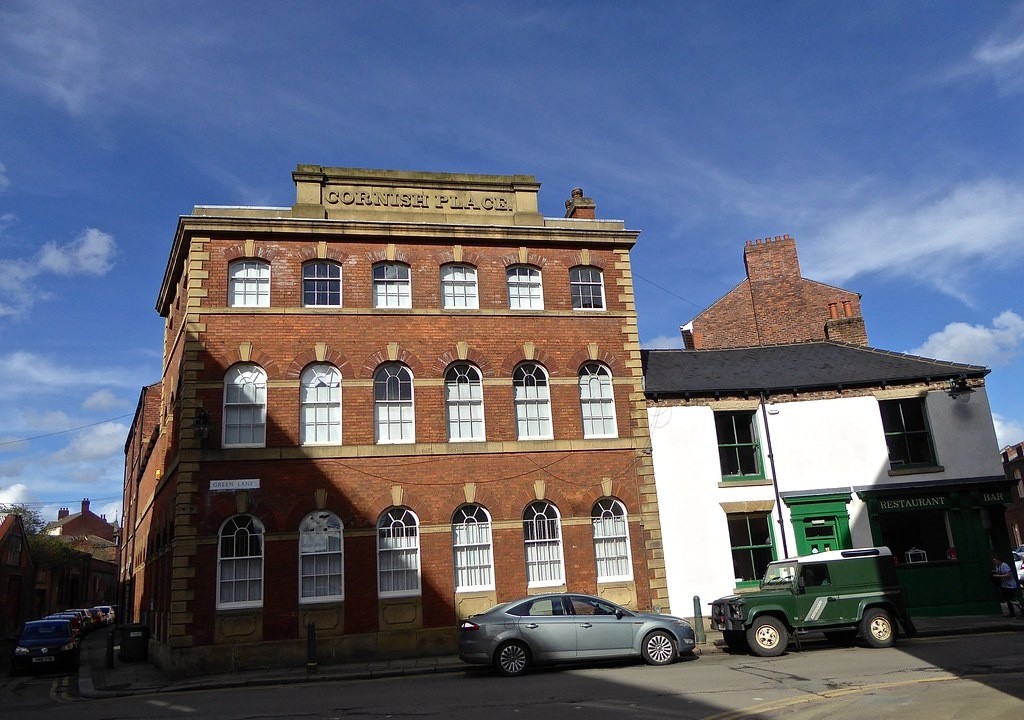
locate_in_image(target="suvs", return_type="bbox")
[708,546,917,657]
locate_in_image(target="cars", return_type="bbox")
[1015,544,1024,559]
[11,604,115,672]
[454,591,698,676]
[1010,551,1024,582]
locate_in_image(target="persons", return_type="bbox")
[992,554,1023,619]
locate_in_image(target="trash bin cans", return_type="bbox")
[118,622,151,663]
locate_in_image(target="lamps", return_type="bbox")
[943,372,977,403]
